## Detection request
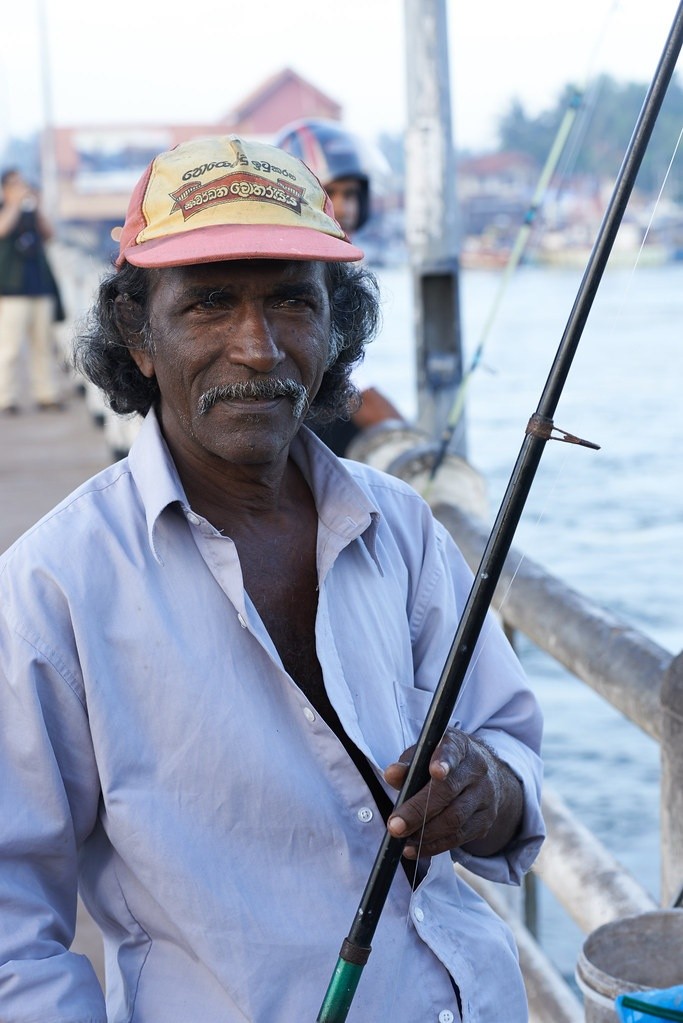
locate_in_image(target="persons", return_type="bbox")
[270,118,404,462]
[0,163,66,416]
[0,132,543,1023]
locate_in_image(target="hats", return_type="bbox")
[114,137,365,269]
[276,121,371,231]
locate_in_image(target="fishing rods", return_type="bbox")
[415,77,598,499]
[310,0,682,1023]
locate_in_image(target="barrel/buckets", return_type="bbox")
[575,907,683,1023]
